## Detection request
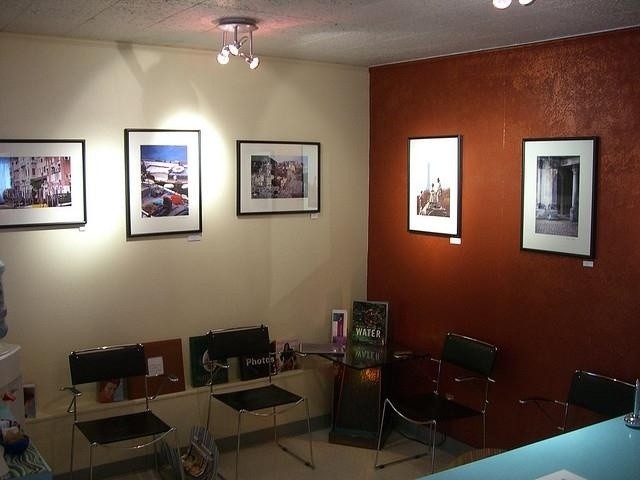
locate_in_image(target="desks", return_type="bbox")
[291,340,431,452]
[416,411,637,479]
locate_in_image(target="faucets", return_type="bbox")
[2,387,18,403]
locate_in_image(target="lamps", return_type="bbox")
[491,0,534,9]
[218,19,259,70]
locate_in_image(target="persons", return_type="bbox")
[97,379,121,404]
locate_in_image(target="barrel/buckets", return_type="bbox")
[0,263,9,339]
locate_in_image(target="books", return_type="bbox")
[179,436,214,480]
[331,308,347,344]
[237,338,278,381]
[188,334,230,387]
[350,300,389,345]
[93,376,129,403]
[274,337,300,369]
[23,383,38,419]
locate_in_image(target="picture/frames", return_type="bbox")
[0,138,87,228]
[237,140,321,216]
[124,130,203,238]
[403,135,599,264]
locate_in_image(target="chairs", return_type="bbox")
[69,343,185,479]
[202,325,316,478]
[373,332,498,474]
[519,367,638,436]
